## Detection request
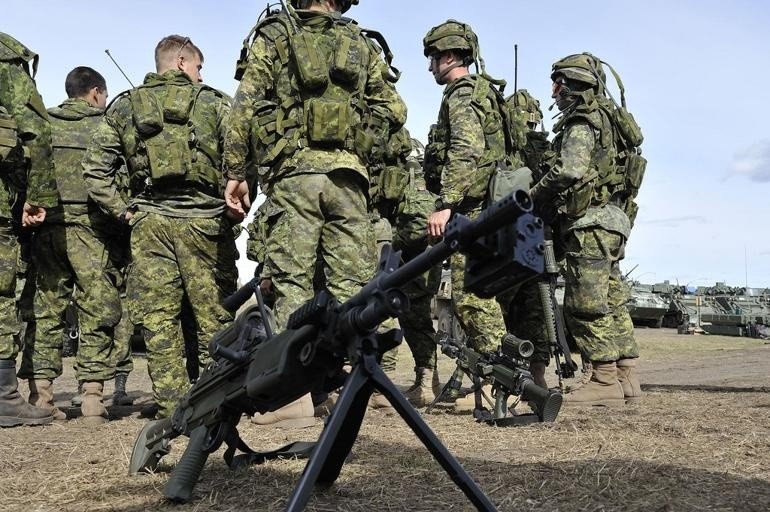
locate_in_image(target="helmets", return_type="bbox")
[424,19,480,62]
[551,53,606,86]
[504,89,541,124]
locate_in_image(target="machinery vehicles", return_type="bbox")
[554,262,770,343]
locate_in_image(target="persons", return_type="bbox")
[1,1,644,426]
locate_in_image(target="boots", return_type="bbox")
[0,358,109,427]
[454,361,548,411]
[251,392,316,429]
[561,357,642,406]
[365,370,397,411]
[112,375,133,406]
[402,366,441,408]
[314,364,352,417]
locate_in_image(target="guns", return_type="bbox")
[128,189,533,512]
[538,238,563,391]
[424,328,563,424]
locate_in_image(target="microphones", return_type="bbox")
[548,99,561,112]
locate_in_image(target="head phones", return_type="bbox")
[558,85,570,97]
